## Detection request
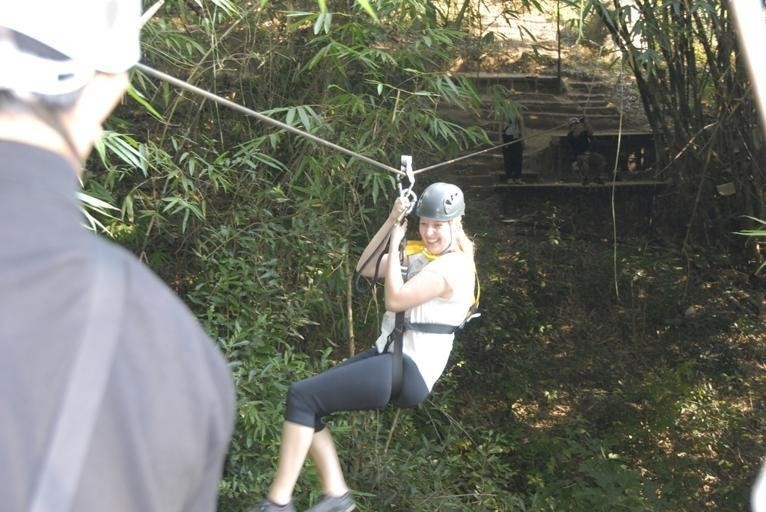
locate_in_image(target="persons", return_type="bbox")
[569,116,610,186]
[0,3,239,512]
[253,183,476,512]
[499,105,531,185]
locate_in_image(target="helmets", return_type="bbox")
[568,117,584,128]
[0,0,142,97]
[415,182,465,221]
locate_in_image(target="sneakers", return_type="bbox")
[303,490,356,512]
[248,495,297,512]
[583,177,603,184]
[508,179,526,185]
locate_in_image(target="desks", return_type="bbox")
[551,132,652,171]
